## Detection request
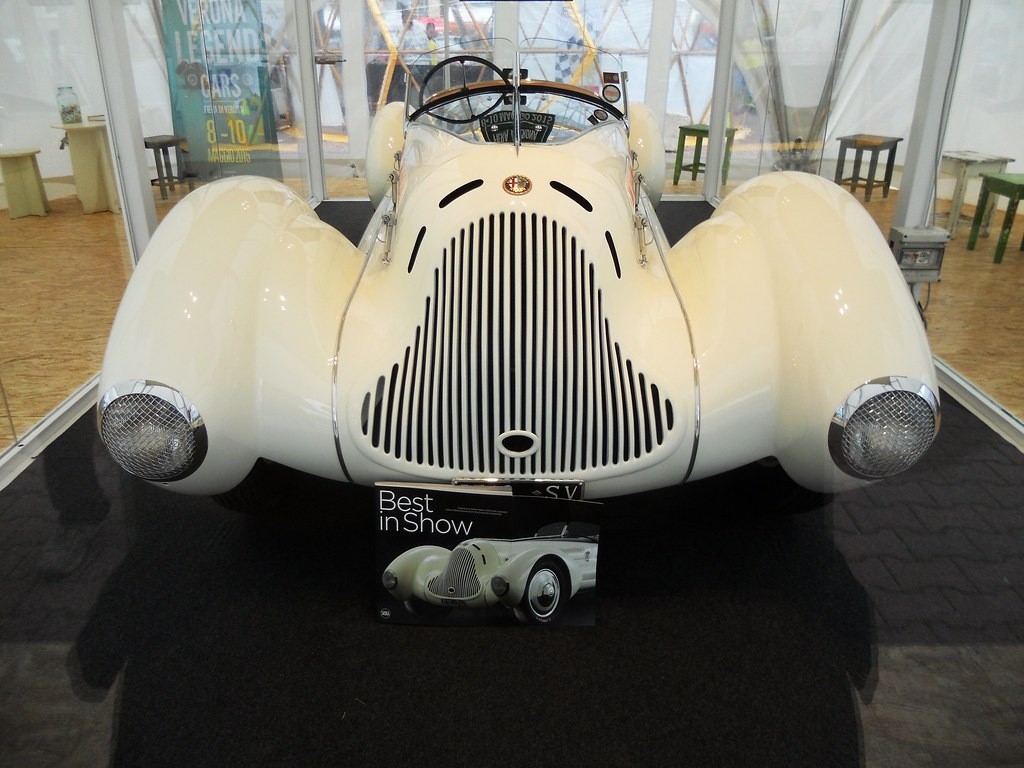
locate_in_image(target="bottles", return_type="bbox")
[56,87,83,124]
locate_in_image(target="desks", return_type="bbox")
[51,122,122,216]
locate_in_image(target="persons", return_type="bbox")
[396,10,431,66]
[426,23,438,66]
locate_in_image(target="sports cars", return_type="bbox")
[96,37,942,500]
[382,522,599,623]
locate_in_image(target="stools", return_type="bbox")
[0,148,51,220]
[144,134,196,199]
[835,134,903,202]
[673,124,738,187]
[930,150,1015,241]
[967,170,1024,264]
